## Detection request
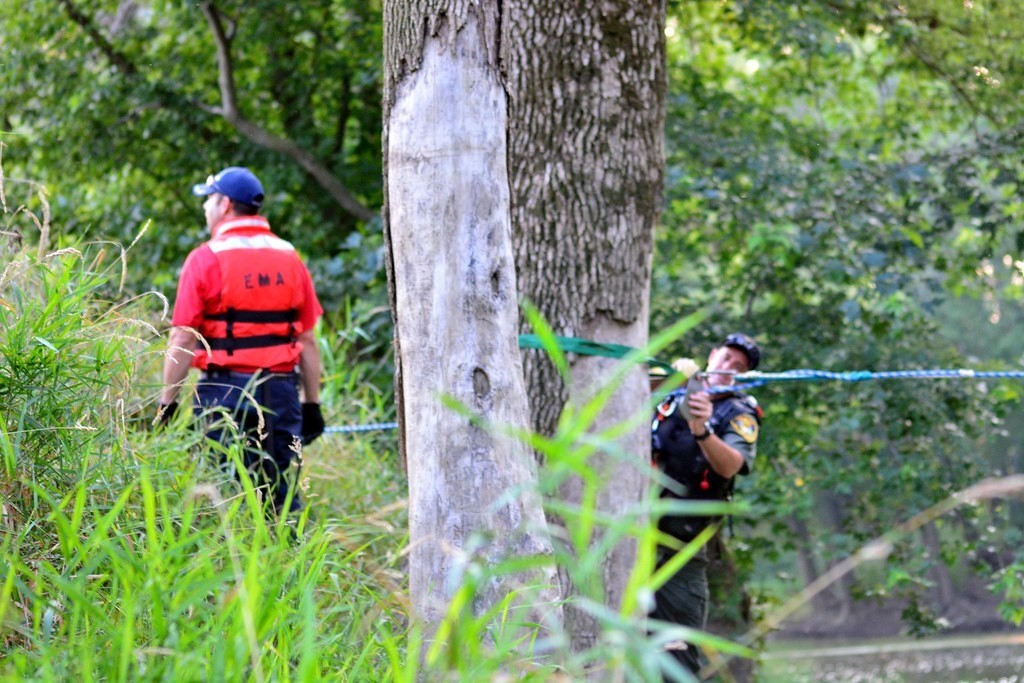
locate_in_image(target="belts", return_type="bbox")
[198,370,290,381]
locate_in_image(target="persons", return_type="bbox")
[153,165,326,542]
[650,331,766,683]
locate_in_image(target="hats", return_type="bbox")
[722,333,760,370]
[192,167,265,208]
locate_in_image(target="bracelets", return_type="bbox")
[692,422,714,442]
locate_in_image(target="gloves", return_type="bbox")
[301,402,325,445]
[161,402,179,431]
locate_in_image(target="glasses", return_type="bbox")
[206,176,233,204]
